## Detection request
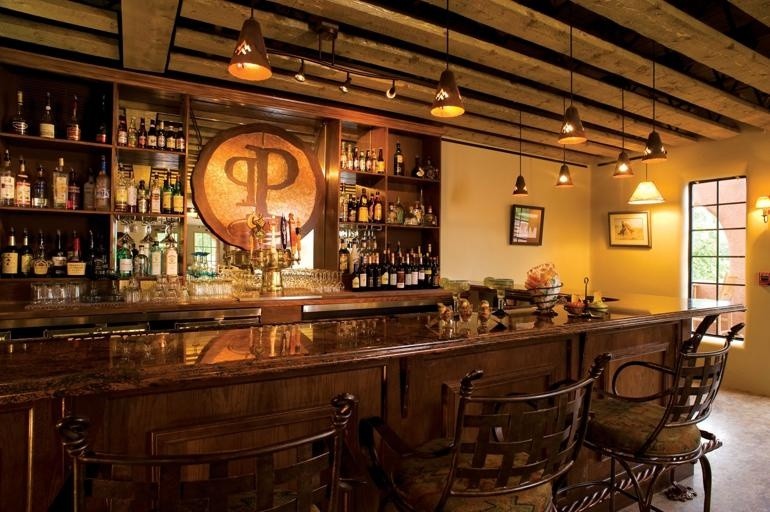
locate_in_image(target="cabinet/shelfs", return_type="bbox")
[117,71,336,332]
[337,111,441,318]
[0,46,116,342]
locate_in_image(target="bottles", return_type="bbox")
[340,182,382,224]
[340,141,385,175]
[340,231,440,290]
[395,142,405,176]
[117,237,178,276]
[337,318,386,350]
[118,162,184,213]
[388,191,436,226]
[413,153,437,180]
[14,87,119,144]
[117,114,186,152]
[3,225,105,276]
[0,148,111,211]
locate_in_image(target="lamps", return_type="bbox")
[627,146,663,204]
[227,1,273,80]
[431,2,463,118]
[341,68,353,93]
[511,107,530,195]
[644,42,666,159]
[387,77,397,98]
[556,3,587,145]
[613,80,635,180]
[556,98,574,188]
[296,57,307,80]
[755,196,770,224]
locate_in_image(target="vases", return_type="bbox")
[530,278,562,311]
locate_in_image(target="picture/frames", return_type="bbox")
[509,203,546,247]
[607,208,653,251]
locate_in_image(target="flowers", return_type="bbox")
[524,262,559,291]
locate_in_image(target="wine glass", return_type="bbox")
[217,265,343,296]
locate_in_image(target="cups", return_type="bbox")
[190,279,234,301]
[29,280,80,303]
[121,277,190,305]
[433,300,491,333]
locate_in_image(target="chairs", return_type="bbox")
[357,350,614,512]
[558,323,747,511]
[674,314,719,351]
[54,391,357,512]
